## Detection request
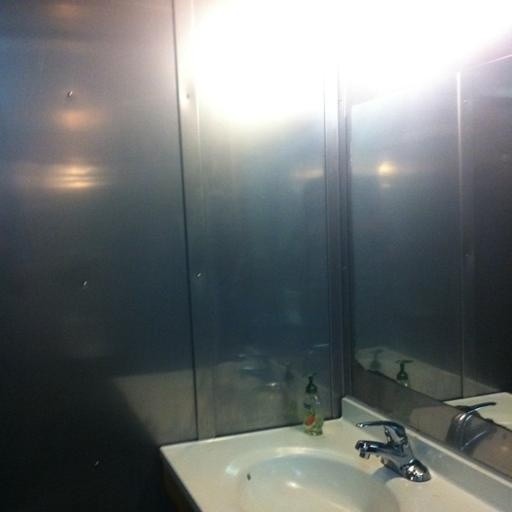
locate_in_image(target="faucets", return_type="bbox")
[354,416,432,483]
[443,400,499,458]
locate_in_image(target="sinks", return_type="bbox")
[235,452,402,512]
